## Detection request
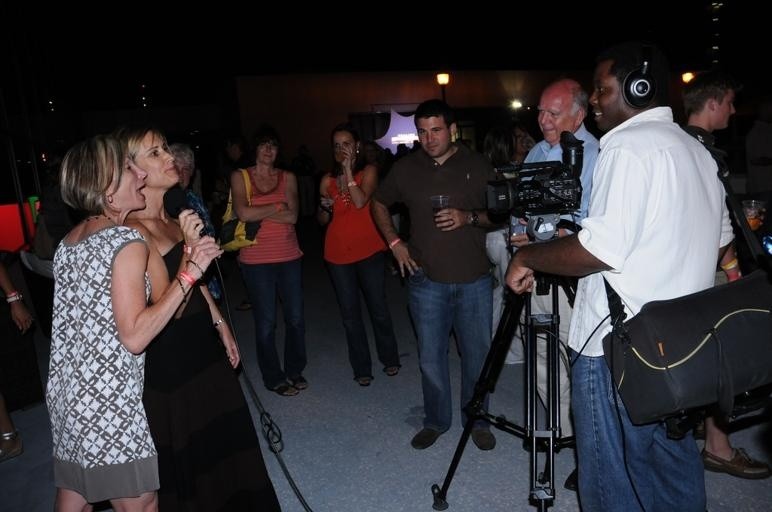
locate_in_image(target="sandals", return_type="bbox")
[357,376,371,386]
[275,383,298,395]
[385,363,399,376]
[291,374,308,389]
[1,430,24,463]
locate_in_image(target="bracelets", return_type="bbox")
[185,260,205,283]
[175,276,187,302]
[721,259,738,271]
[6,295,24,303]
[348,181,357,187]
[389,238,401,248]
[6,291,17,297]
[212,318,224,327]
[179,271,195,284]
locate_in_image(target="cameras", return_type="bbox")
[488,129,583,216]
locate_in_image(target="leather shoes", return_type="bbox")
[472,428,495,450]
[235,302,252,311]
[702,447,772,478]
[411,428,440,448]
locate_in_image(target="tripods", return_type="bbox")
[431,219,582,511]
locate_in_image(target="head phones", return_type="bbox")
[621,52,656,110]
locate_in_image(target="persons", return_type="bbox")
[506,124,535,164]
[216,125,314,397]
[45,132,224,512]
[316,125,401,386]
[681,71,771,478]
[94,122,282,512]
[508,42,735,512]
[220,134,254,311]
[510,79,600,492]
[0,260,31,460]
[482,128,531,366]
[371,98,514,449]
[161,143,223,302]
[365,142,398,276]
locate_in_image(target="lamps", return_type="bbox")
[436,71,453,101]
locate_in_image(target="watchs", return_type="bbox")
[471,210,479,226]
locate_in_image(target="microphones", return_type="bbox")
[164,187,201,232]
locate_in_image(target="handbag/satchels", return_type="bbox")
[218,168,262,251]
